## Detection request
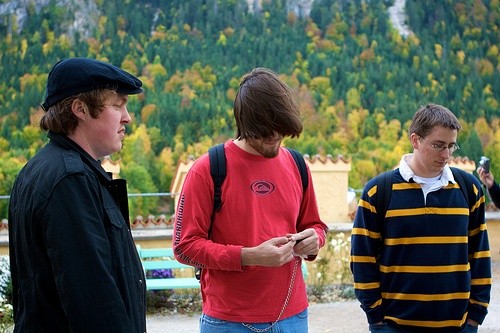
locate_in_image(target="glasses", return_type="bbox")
[418,133,460,152]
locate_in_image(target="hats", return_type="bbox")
[41,56,144,111]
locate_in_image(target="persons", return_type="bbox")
[172,66,329,333]
[349,103,492,333]
[7,57,147,333]
[476,165,500,210]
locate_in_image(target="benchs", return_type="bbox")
[138,249,201,291]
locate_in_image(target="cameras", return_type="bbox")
[478,156,490,174]
